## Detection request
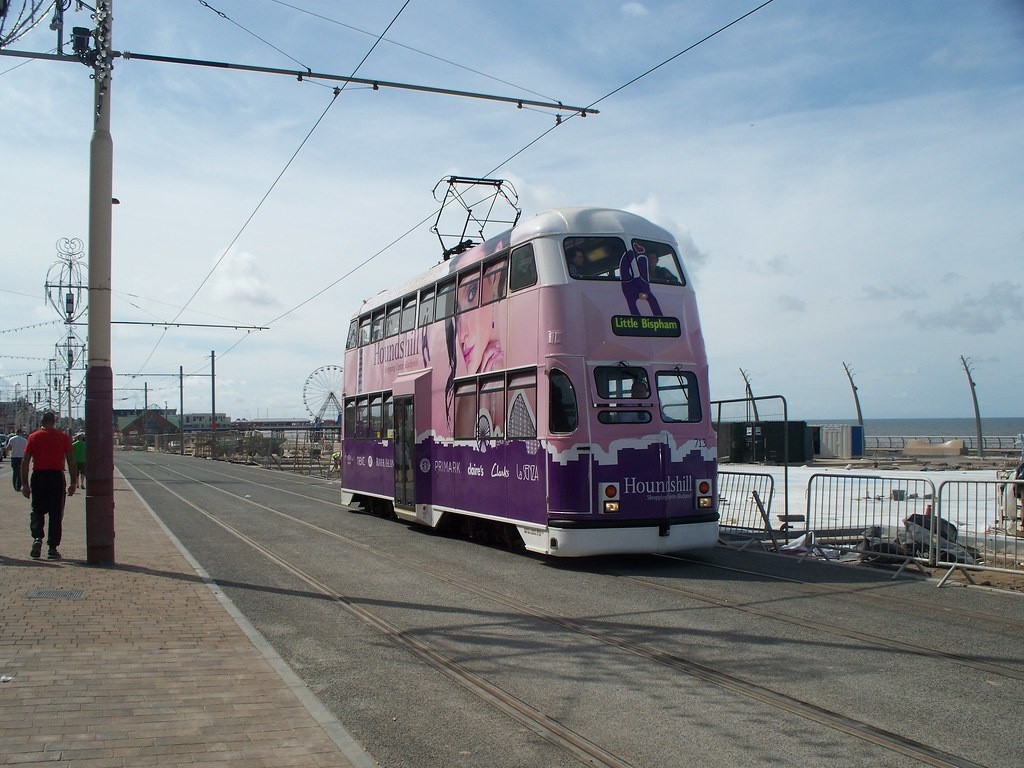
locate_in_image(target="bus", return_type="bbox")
[339,176,722,564]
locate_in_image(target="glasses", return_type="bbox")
[632,390,643,394]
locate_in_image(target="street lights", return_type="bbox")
[26,374,32,434]
[48,358,56,410]
[14,383,21,435]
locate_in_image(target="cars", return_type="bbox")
[168,440,180,450]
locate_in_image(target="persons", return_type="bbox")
[20,412,77,558]
[5,429,27,492]
[445,226,536,441]
[71,434,85,489]
[568,247,594,280]
[646,247,679,284]
[6,431,28,450]
[616,381,676,422]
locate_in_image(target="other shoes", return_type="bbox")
[46,549,62,558]
[30,539,42,557]
